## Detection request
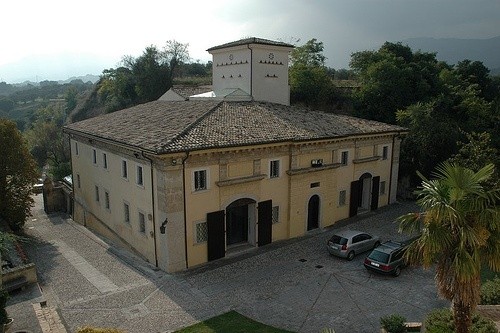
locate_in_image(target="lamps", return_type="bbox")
[159,218,168,235]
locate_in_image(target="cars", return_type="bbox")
[364,239,417,277]
[32,179,44,193]
[327,231,380,261]
